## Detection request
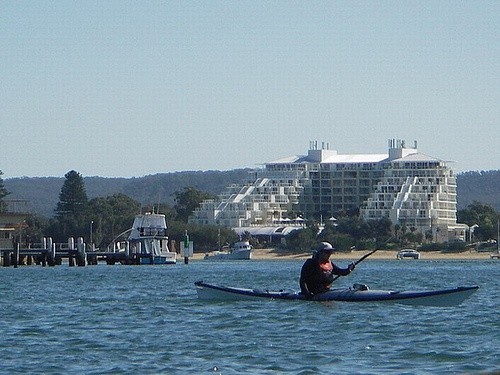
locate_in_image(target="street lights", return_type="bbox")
[20,220,26,248]
[90,221,94,252]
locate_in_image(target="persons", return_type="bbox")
[300,241,356,297]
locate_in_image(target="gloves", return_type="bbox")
[306,291,314,298]
[347,262,355,274]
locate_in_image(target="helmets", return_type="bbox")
[316,242,335,252]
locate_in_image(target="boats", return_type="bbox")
[193,279,480,308]
[116,202,177,265]
[203,241,254,260]
